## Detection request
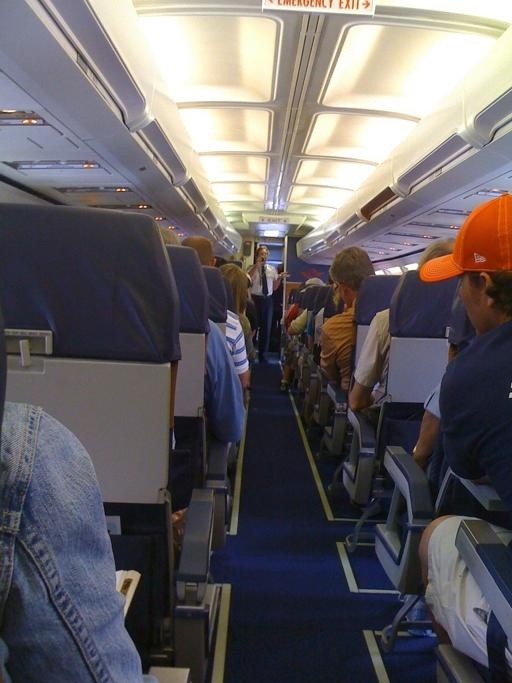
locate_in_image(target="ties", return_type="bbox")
[261,266,268,296]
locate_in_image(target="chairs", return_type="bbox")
[0,200,256,682]
[285,270,512,683]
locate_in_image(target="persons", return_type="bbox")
[245,245,290,365]
[161,228,257,461]
[1,401,158,682]
[281,237,455,493]
[419,193,511,675]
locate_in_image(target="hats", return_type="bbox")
[419,193,512,283]
[305,277,322,286]
